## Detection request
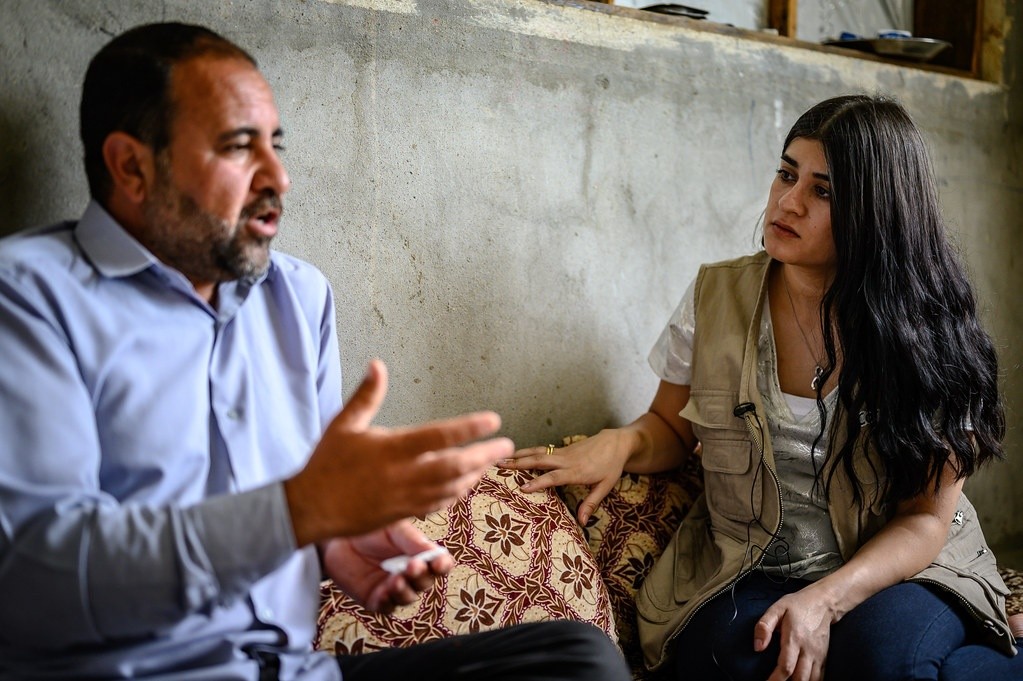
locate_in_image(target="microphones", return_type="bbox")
[734,402,757,417]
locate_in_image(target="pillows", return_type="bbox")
[550,433,706,674]
[311,455,624,650]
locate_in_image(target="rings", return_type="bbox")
[546,443,555,455]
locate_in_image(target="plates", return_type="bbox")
[822,38,951,61]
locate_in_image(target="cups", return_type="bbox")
[879,29,912,37]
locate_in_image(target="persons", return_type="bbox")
[497,93,1023,681]
[0,20,517,681]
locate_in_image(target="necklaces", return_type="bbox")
[779,265,841,390]
